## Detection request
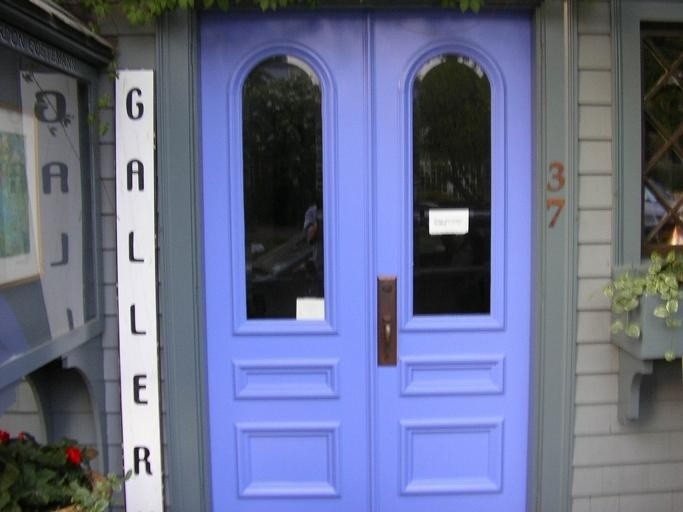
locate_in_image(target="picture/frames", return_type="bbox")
[0,99,44,291]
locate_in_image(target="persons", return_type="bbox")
[303,190,323,299]
[249,142,321,318]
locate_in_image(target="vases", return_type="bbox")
[46,472,115,512]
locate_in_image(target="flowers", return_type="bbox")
[0,428,83,466]
